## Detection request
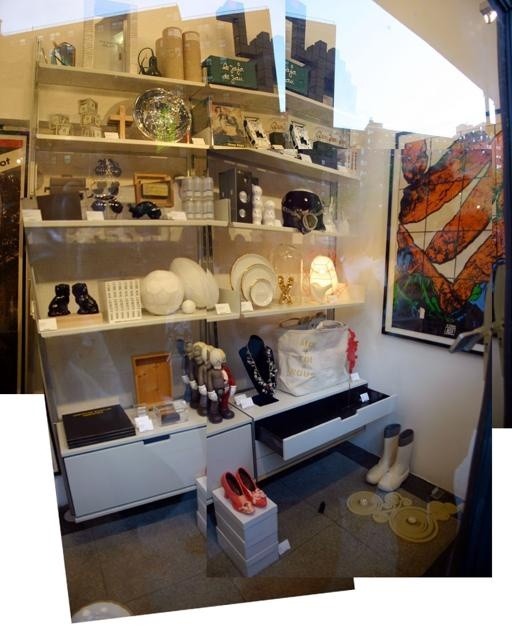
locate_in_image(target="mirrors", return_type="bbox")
[83,0,138,75]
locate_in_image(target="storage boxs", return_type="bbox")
[201,54,258,91]
[212,487,280,579]
[286,56,310,95]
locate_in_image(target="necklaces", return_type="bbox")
[245,345,278,395]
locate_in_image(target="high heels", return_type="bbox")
[221,471,255,516]
[236,466,267,508]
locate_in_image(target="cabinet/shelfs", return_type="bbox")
[22,59,394,524]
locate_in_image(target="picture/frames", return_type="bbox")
[133,171,175,208]
[380,107,505,357]
[0,131,29,395]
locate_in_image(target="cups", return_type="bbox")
[182,176,215,220]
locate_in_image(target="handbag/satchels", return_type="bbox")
[272,317,350,396]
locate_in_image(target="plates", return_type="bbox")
[241,263,277,305]
[249,278,274,307]
[230,253,274,303]
[132,86,194,146]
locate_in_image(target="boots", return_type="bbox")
[377,429,413,492]
[366,424,401,485]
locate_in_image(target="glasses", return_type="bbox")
[91,157,123,214]
